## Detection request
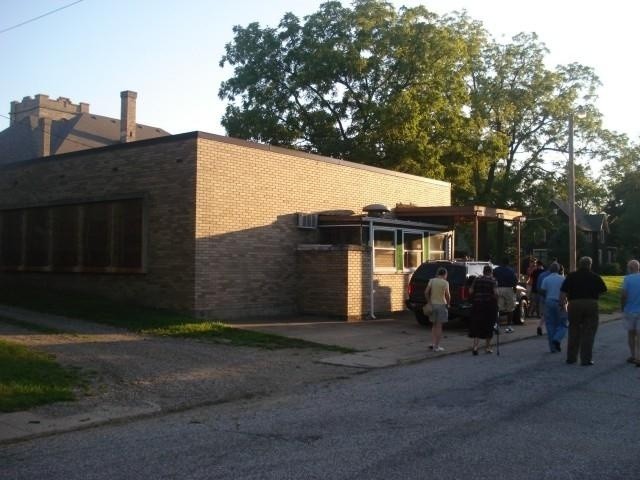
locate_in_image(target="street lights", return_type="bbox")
[567,104,587,274]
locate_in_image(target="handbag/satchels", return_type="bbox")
[423,303,432,315]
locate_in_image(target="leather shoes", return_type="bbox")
[472,346,493,354]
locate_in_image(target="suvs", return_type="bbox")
[406,256,528,327]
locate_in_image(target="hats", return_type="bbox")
[550,263,560,273]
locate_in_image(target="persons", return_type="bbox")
[541,262,567,354]
[493,255,517,333]
[535,262,558,336]
[422,267,451,353]
[620,259,640,368]
[469,264,500,356]
[528,260,544,317]
[559,256,609,367]
[526,258,536,295]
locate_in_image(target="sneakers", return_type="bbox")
[581,361,594,366]
[538,327,542,335]
[552,339,561,352]
[429,343,444,352]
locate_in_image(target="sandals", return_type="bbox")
[627,357,640,366]
